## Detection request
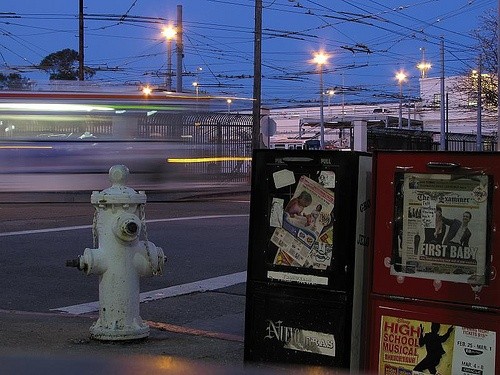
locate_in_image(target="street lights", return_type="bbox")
[225,97,233,113]
[417,48,431,77]
[161,23,180,91]
[313,53,332,149]
[395,69,406,128]
[323,89,335,108]
[192,82,201,94]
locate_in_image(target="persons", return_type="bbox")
[294,204,322,229]
[432,207,444,239]
[440,215,472,248]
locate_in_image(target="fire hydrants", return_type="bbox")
[65,164,167,344]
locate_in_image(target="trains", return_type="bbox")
[0,79,212,192]
[184,141,321,177]
